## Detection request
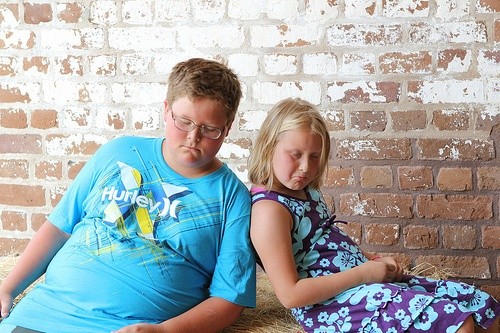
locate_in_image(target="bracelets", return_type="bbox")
[371,255,381,260]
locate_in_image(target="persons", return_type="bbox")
[249,97,499,333]
[0,59,256,332]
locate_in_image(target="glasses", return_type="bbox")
[171,108,225,140]
